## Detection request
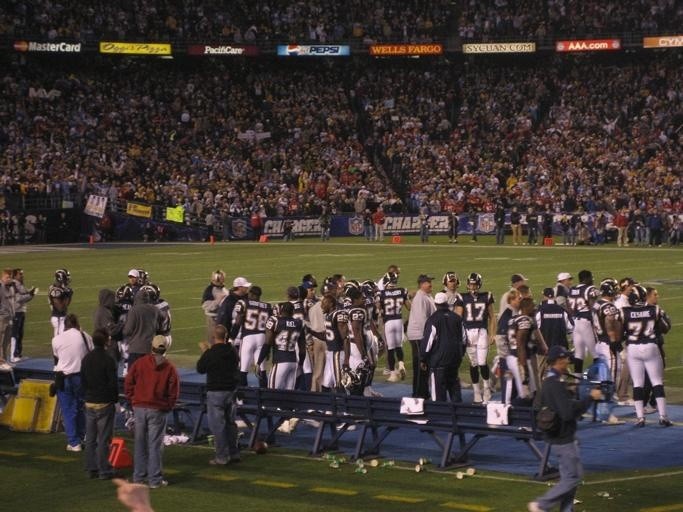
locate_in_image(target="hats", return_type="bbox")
[297,286,307,299]
[542,288,554,298]
[250,286,261,295]
[233,277,251,287]
[279,302,294,315]
[418,274,435,282]
[557,273,572,281]
[128,269,139,277]
[302,280,313,288]
[511,274,528,282]
[435,292,448,304]
[621,278,638,287]
[548,345,575,360]
[153,335,166,350]
[289,287,298,298]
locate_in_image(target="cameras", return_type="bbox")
[597,377,614,401]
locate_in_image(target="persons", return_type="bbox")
[196,325,241,464]
[527,345,602,511]
[95,268,172,376]
[1,267,73,369]
[80,329,119,480]
[112,478,151,512]
[1,1,682,248]
[125,335,180,488]
[51,313,93,452]
[201,266,673,428]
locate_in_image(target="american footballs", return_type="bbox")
[254,441,268,453]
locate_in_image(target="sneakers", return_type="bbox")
[659,415,673,426]
[602,414,625,424]
[209,457,227,465]
[386,376,398,383]
[66,445,81,452]
[483,389,491,402]
[150,480,168,489]
[231,453,240,462]
[474,393,482,402]
[88,469,99,479]
[133,480,147,484]
[633,418,645,428]
[643,406,658,414]
[99,472,122,479]
[383,369,399,375]
[618,399,635,406]
[398,365,405,380]
[527,501,546,512]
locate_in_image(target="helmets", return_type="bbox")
[356,364,373,385]
[628,286,647,306]
[303,274,317,287]
[443,272,460,288]
[345,280,362,297]
[600,278,619,297]
[324,277,334,288]
[341,367,358,389]
[117,285,133,299]
[211,270,224,286]
[466,273,482,290]
[320,285,334,297]
[383,271,398,286]
[56,269,72,285]
[140,285,161,303]
[138,271,152,285]
[361,280,381,302]
